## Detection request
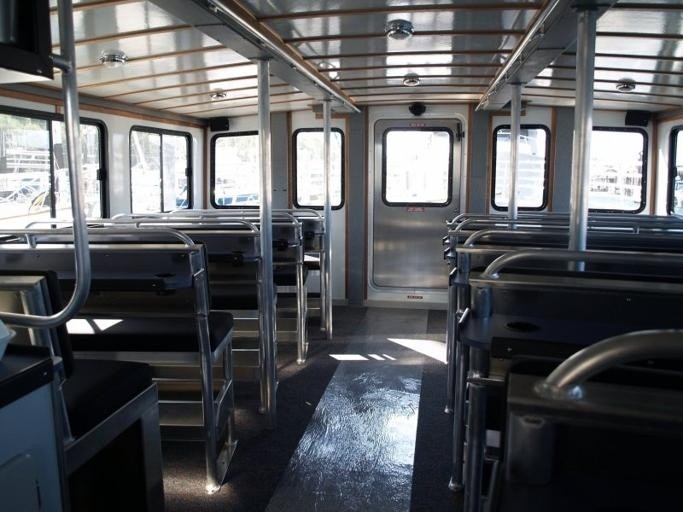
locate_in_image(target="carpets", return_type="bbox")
[412,308,492,510]
[130,303,367,512]
